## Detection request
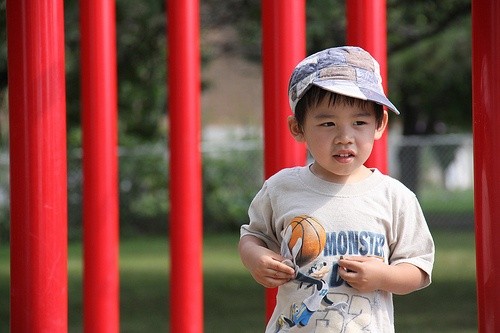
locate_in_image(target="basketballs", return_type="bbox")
[286,214,327,263]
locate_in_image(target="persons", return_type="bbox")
[239,46,436,333]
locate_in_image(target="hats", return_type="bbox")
[288,46,400,116]
